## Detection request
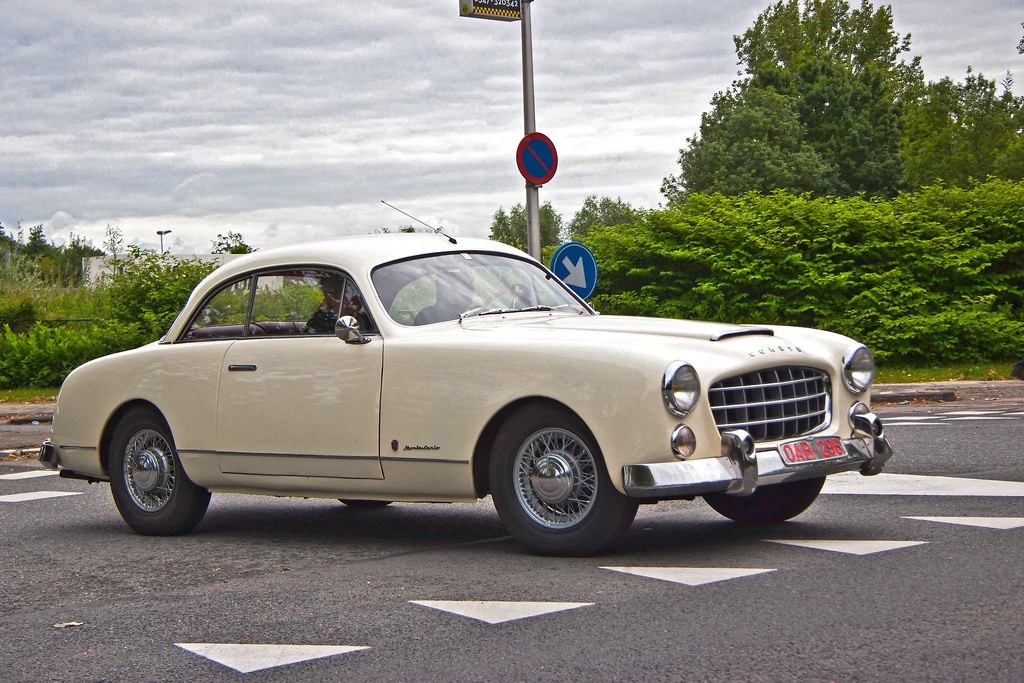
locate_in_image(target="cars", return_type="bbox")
[38,232,897,556]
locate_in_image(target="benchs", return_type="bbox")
[188,321,309,340]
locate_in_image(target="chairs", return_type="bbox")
[413,306,433,327]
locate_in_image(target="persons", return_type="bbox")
[303,272,372,334]
[413,260,476,327]
